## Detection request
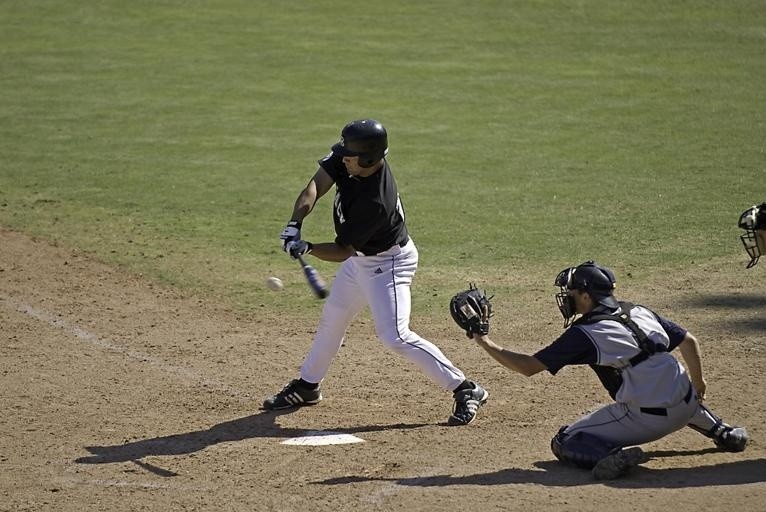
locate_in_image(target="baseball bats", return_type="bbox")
[298,253,330,300]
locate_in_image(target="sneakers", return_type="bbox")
[724,426,747,452]
[263,378,322,410]
[592,446,643,481]
[448,381,489,426]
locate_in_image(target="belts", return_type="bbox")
[640,381,692,416]
[352,235,408,256]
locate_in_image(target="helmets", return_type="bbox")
[554,260,619,328]
[331,119,389,168]
[738,203,766,269]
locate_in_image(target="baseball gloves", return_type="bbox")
[451,290,492,338]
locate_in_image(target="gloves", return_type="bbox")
[280,219,313,261]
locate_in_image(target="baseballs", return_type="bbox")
[267,277,284,292]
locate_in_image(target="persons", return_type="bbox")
[254,117,491,428]
[448,259,750,482]
